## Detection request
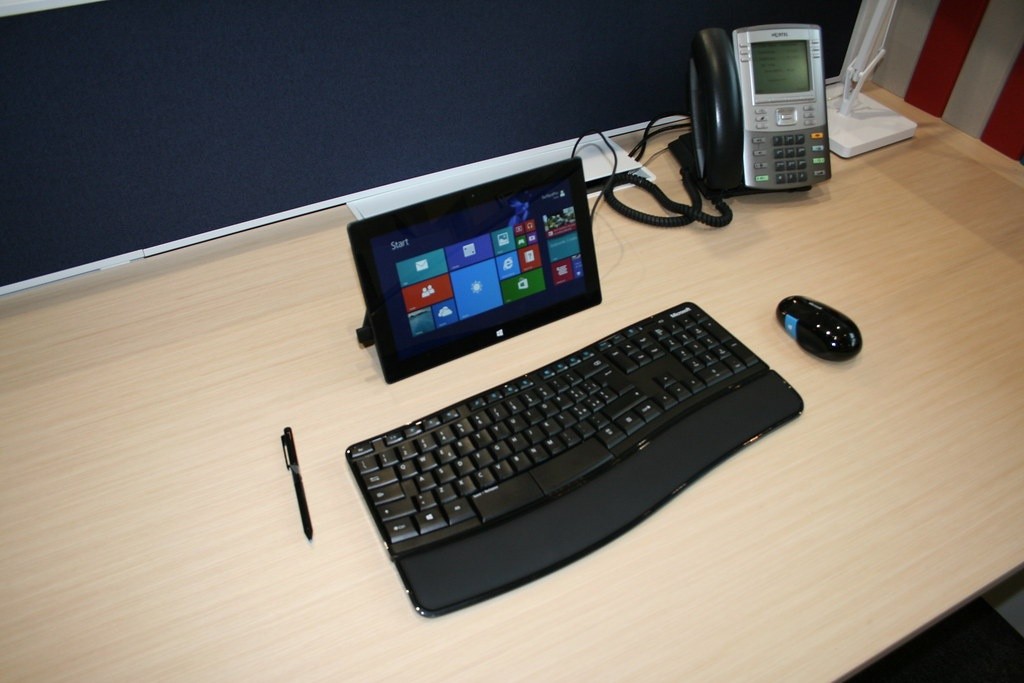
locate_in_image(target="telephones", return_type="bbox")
[689,22,832,200]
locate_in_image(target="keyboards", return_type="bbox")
[345,301,804,618]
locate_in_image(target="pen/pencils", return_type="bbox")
[280,427,314,545]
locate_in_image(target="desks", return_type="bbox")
[0,80,1024,683]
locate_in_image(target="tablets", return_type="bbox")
[347,156,602,384]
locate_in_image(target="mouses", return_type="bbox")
[775,295,863,362]
